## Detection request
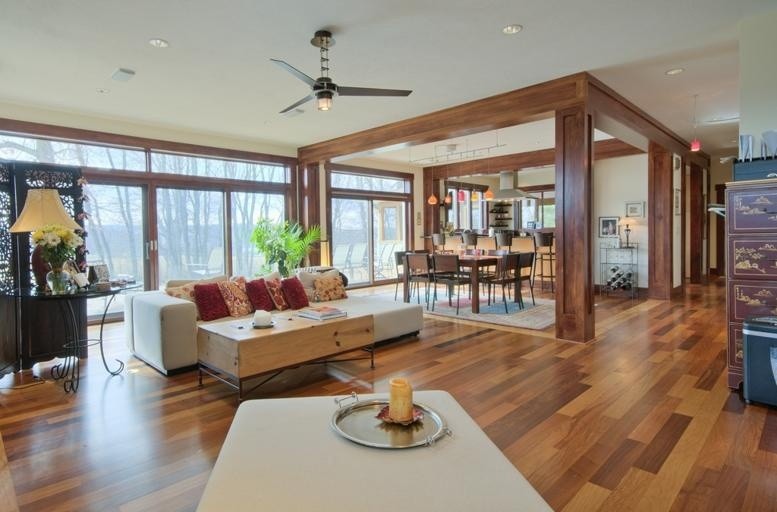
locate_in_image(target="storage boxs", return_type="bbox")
[741,314,777,408]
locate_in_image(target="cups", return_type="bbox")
[448,222,454,232]
[254,310,271,325]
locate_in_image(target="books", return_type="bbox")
[295,306,347,321]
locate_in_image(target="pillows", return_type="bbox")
[162,267,348,322]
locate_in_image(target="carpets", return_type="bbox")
[377,285,599,331]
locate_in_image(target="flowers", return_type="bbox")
[31,224,84,295]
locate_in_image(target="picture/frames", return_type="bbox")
[624,201,645,218]
[598,216,620,238]
[674,188,682,215]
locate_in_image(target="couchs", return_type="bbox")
[123,263,425,377]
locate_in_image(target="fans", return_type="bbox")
[269,30,413,113]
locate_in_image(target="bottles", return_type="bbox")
[489,225,494,237]
[607,266,631,290]
[527,222,534,229]
[534,224,536,229]
[389,377,413,421]
[617,236,622,248]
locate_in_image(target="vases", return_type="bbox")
[45,260,70,295]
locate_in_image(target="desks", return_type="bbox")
[5,277,144,394]
[194,391,552,512]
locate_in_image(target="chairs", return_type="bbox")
[394,232,537,315]
[532,231,555,294]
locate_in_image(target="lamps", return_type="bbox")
[427,149,495,205]
[690,94,700,152]
[616,214,640,248]
[8,188,84,292]
[316,91,333,113]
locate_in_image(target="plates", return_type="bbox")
[252,321,274,328]
[375,403,424,425]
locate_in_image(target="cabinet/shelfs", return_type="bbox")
[598,242,639,299]
[488,202,512,227]
[724,186,776,389]
[334,240,396,278]
